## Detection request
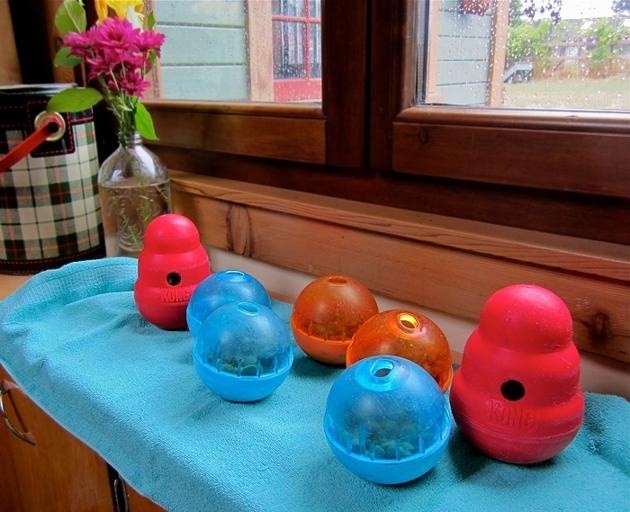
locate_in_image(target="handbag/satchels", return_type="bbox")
[0,80,107,278]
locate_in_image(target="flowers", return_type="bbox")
[49,0,166,243]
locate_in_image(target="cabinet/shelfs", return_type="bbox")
[0,365,165,512]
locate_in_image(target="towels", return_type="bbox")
[0,257,629,512]
